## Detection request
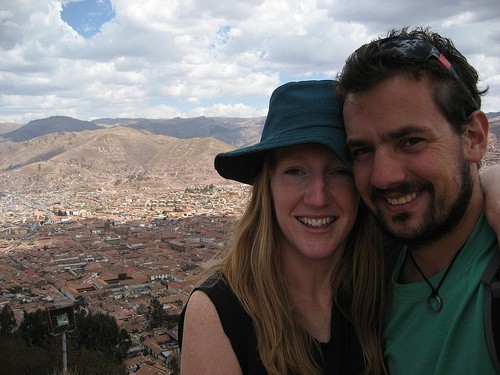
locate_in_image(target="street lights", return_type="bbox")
[45,302,78,375]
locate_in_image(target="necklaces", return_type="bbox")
[400,219,474,317]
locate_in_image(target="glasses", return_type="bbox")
[344,36,481,110]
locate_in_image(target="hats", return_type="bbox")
[214,80,353,186]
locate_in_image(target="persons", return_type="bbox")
[335,26,500,375]
[178,80,500,375]
